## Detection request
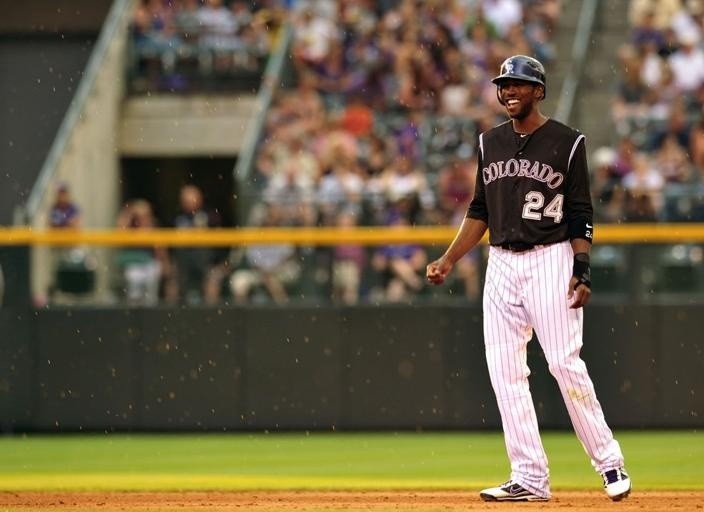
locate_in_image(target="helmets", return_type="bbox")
[491,54,546,87]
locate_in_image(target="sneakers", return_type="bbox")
[602,464,632,501]
[478,479,552,503]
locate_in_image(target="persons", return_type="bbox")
[103,2,704,306]
[43,183,81,266]
[425,52,636,503]
